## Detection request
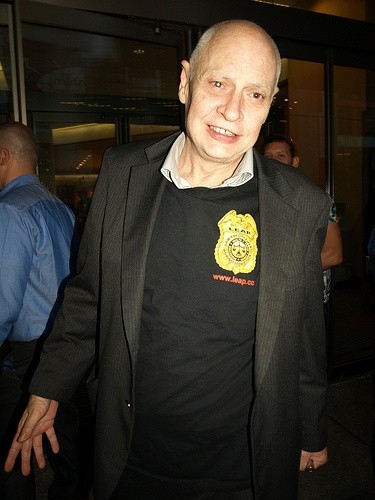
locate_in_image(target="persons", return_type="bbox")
[0,18,342,500]
